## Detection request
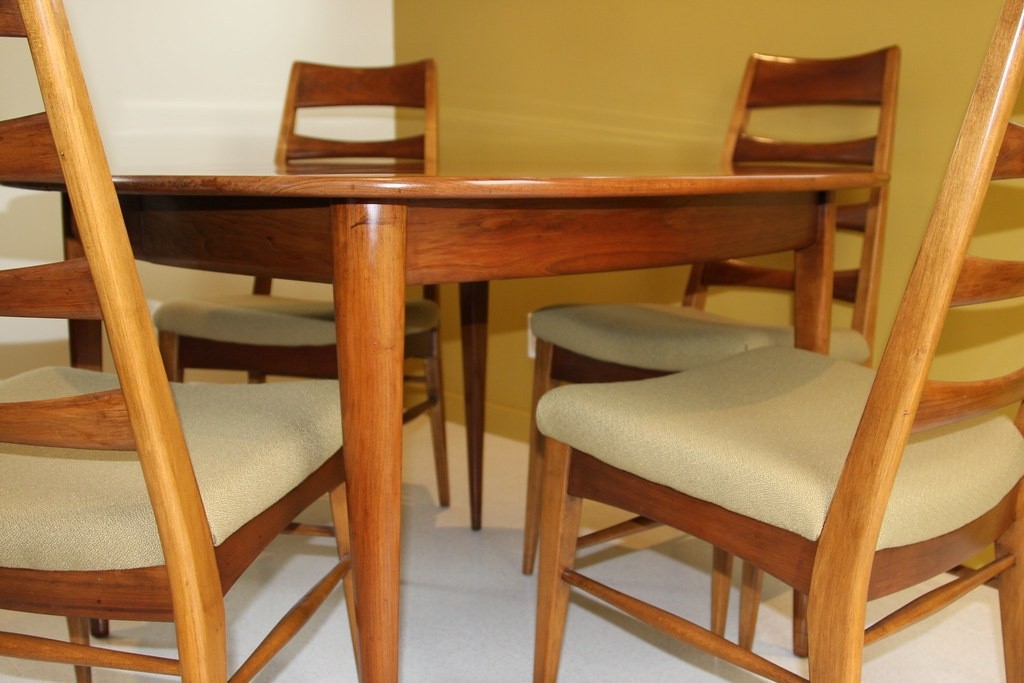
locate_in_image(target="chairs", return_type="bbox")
[0,0,358,683]
[151,60,448,551]
[532,0,1024,683]
[523,44,902,642]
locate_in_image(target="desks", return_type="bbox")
[49,166,891,683]
